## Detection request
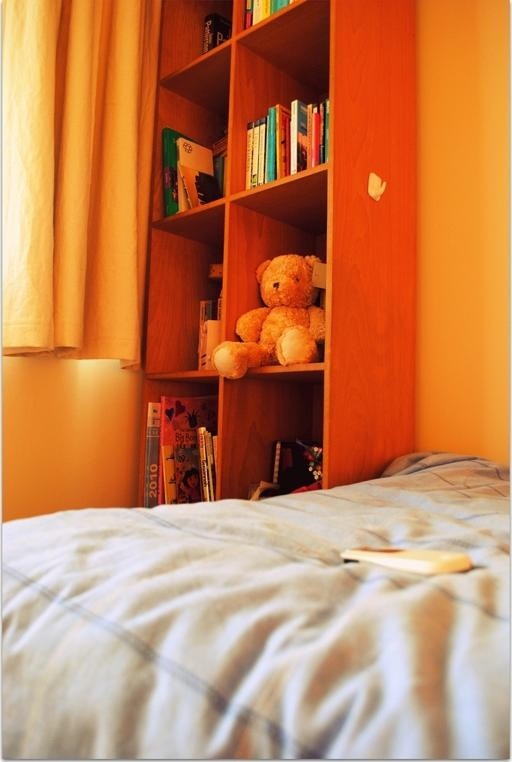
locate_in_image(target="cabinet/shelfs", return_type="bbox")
[138,0,417,508]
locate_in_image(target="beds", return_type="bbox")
[1,456,511,759]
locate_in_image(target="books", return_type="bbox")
[162,124,228,219]
[244,0,294,30]
[202,14,232,56]
[245,99,329,191]
[142,394,219,508]
[196,299,223,369]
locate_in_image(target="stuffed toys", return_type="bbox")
[209,252,327,380]
